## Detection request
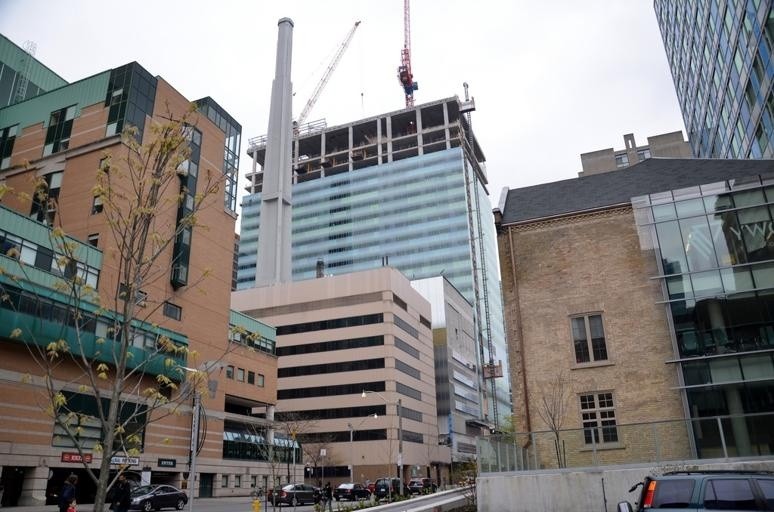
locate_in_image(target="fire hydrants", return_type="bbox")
[251,498,263,512]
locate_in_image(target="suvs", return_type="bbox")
[372,477,412,503]
[617,464,774,512]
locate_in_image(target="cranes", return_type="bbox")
[394,0,422,111]
[292,19,366,139]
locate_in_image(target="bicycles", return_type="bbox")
[249,484,266,501]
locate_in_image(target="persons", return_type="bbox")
[108,474,132,512]
[249,483,256,499]
[441,477,446,491]
[67,497,78,512]
[257,487,263,500]
[310,481,333,512]
[366,478,370,486]
[56,474,78,512]
[431,480,437,493]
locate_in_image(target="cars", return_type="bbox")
[406,478,440,496]
[266,478,335,508]
[47,486,62,504]
[130,484,190,511]
[459,476,475,487]
[367,481,376,496]
[332,481,374,503]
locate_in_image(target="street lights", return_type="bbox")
[361,385,406,499]
[346,411,381,487]
[168,360,204,511]
